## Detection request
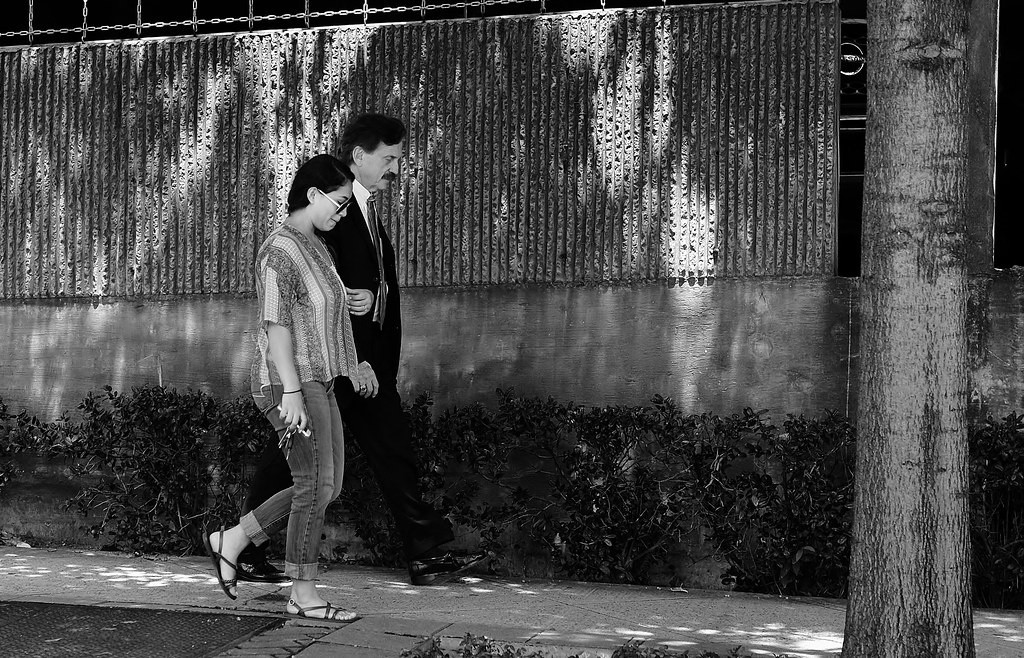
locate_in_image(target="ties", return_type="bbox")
[367,196,386,331]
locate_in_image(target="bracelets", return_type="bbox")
[282,388,302,394]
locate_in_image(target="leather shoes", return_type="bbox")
[235,556,291,583]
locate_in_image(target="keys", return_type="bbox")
[278,425,297,460]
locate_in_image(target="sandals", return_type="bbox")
[283,597,357,623]
[202,530,238,600]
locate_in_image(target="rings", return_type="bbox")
[360,386,366,391]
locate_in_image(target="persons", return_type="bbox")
[203,154,375,622]
[235,113,491,587]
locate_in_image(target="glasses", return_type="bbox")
[317,188,350,214]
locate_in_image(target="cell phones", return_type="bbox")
[277,403,312,437]
